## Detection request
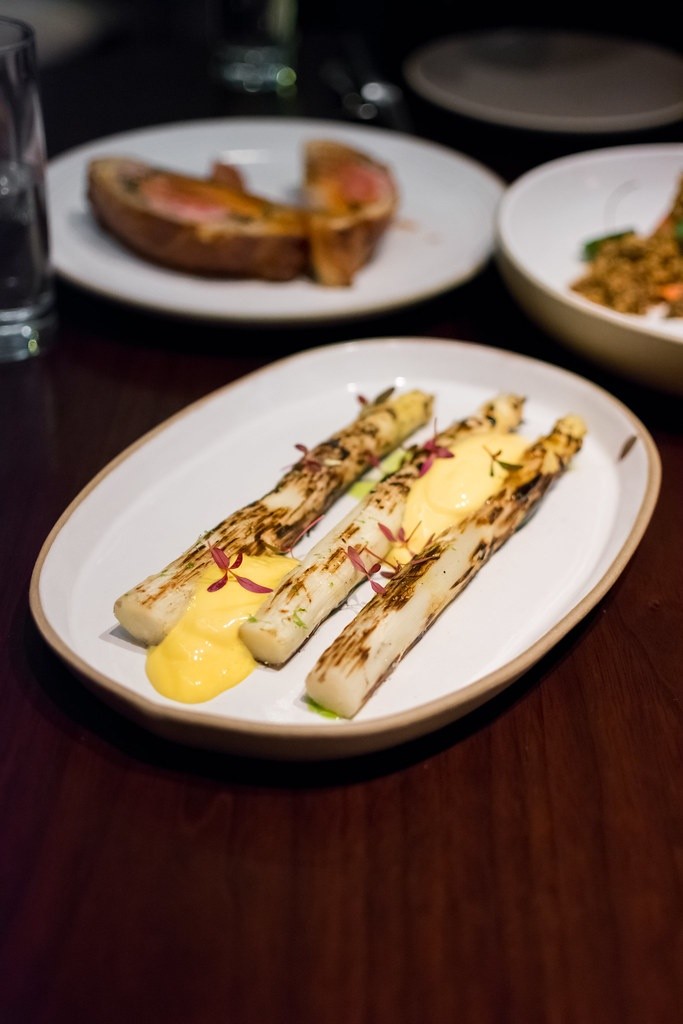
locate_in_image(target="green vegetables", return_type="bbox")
[582,221,683,262]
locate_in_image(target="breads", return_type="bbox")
[304,142,396,286]
[87,159,309,282]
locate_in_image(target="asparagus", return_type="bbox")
[110,387,584,722]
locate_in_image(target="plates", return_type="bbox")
[27,336,662,759]
[408,30,682,135]
[47,114,508,320]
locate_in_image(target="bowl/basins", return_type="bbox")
[496,142,682,394]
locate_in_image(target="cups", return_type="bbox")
[0,16,56,361]
